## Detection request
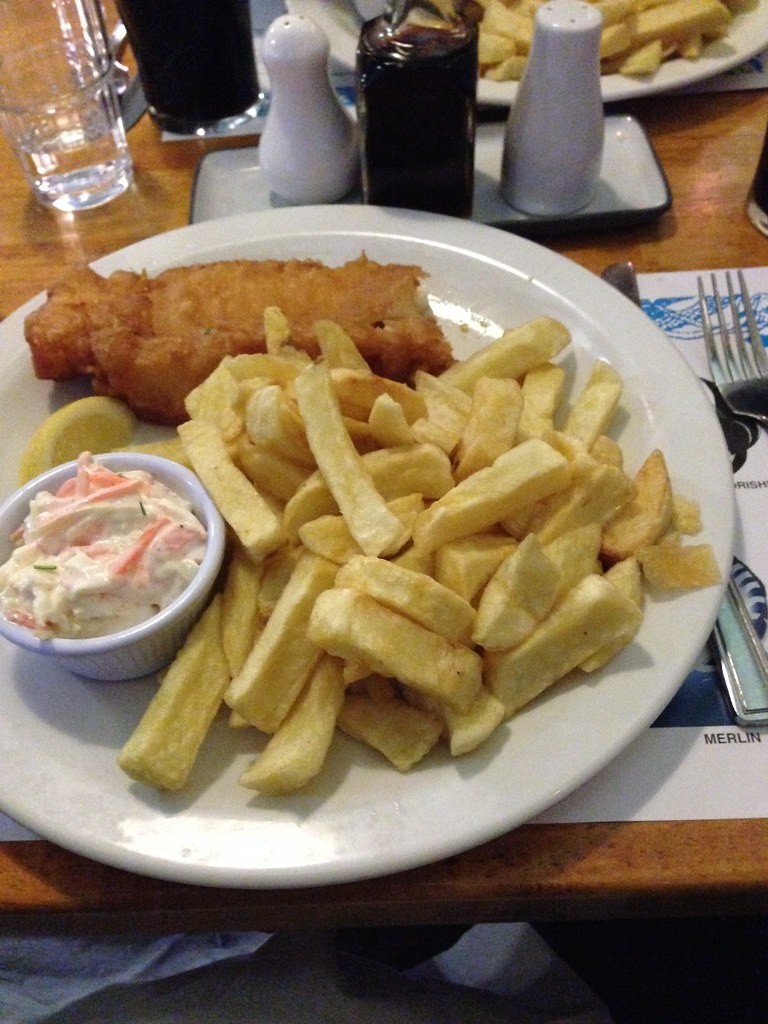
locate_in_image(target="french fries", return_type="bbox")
[476,0,757,79]
[93,305,723,795]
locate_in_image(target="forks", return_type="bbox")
[697,269,768,421]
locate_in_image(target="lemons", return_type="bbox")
[18,395,138,493]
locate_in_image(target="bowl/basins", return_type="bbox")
[0,455,225,682]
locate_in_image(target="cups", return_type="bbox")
[114,0,263,136]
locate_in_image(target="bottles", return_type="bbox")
[501,1,605,218]
[0,0,132,211]
[358,1,478,217]
[258,13,359,205]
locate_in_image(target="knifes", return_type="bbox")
[603,260,768,726]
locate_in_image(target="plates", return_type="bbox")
[0,205,734,892]
[284,0,768,106]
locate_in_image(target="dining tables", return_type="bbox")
[0,0,768,936]
